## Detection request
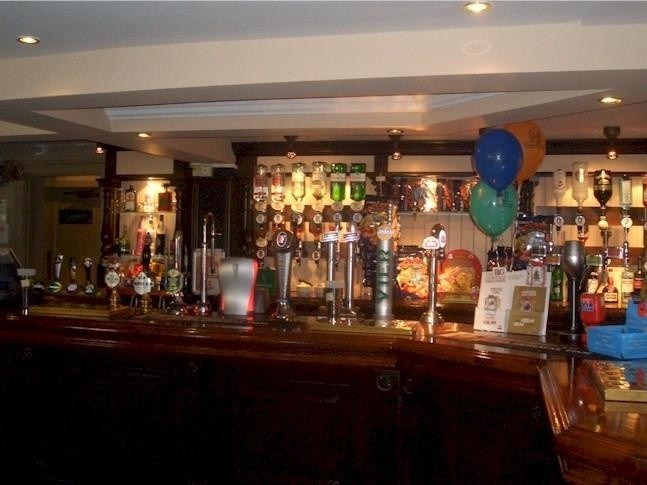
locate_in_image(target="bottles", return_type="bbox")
[602,255,646,304]
[136,214,167,256]
[50,251,96,299]
[551,264,563,301]
[254,213,365,254]
[124,181,173,213]
[552,162,647,215]
[118,224,131,255]
[252,162,368,206]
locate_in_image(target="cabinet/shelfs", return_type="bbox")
[120,175,184,258]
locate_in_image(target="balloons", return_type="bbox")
[468,181,518,242]
[472,127,523,198]
[503,121,545,186]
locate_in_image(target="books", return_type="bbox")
[584,358,647,405]
[473,267,546,336]
